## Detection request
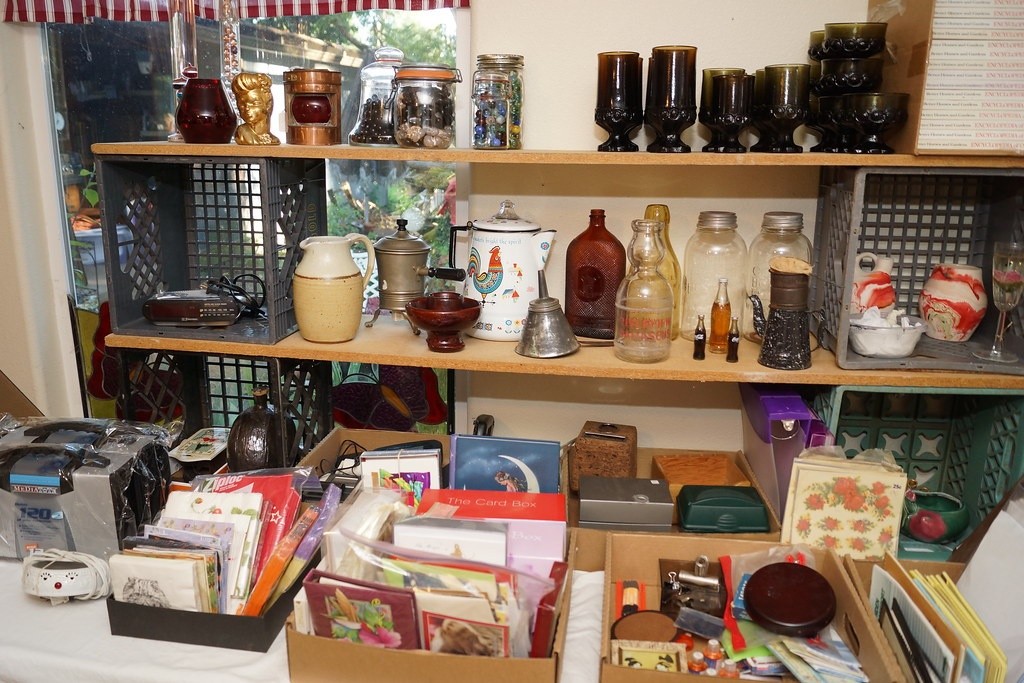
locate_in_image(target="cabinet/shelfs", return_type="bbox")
[89,145,1024,390]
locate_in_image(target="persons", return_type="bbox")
[231,72,282,146]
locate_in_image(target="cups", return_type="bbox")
[374,219,466,311]
[174,79,238,144]
[917,263,988,342]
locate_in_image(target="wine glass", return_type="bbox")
[698,64,810,152]
[973,242,1024,363]
[808,22,910,153]
[595,23,697,152]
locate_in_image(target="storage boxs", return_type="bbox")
[107,0,1024,683]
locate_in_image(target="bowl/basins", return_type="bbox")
[850,312,928,358]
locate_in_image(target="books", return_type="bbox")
[107,461,344,616]
[777,450,1024,683]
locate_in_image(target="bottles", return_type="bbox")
[470,54,524,151]
[349,47,404,147]
[384,60,462,149]
[566,203,814,363]
[226,387,297,472]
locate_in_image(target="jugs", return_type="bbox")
[852,252,896,315]
[449,200,557,340]
[293,233,375,343]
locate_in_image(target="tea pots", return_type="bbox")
[748,256,826,370]
